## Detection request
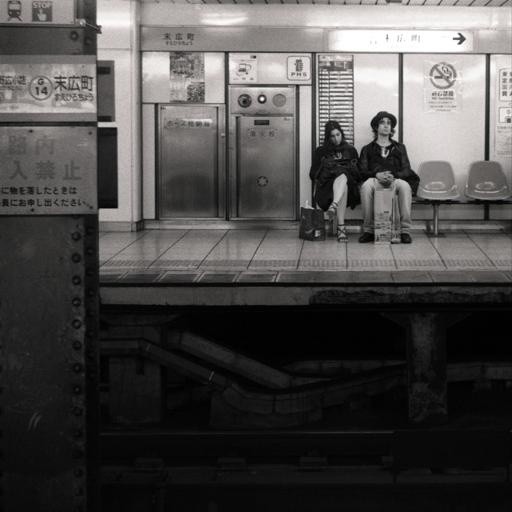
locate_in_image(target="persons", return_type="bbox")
[308,120,362,245]
[356,111,421,245]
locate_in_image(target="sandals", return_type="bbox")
[336,223,350,244]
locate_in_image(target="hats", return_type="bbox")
[370,110,397,129]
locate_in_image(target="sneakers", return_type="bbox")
[399,233,413,244]
[358,231,373,244]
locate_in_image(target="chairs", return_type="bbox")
[414,160,511,238]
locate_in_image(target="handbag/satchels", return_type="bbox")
[371,180,404,246]
[297,199,328,242]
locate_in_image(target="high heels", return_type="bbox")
[327,201,340,223]
